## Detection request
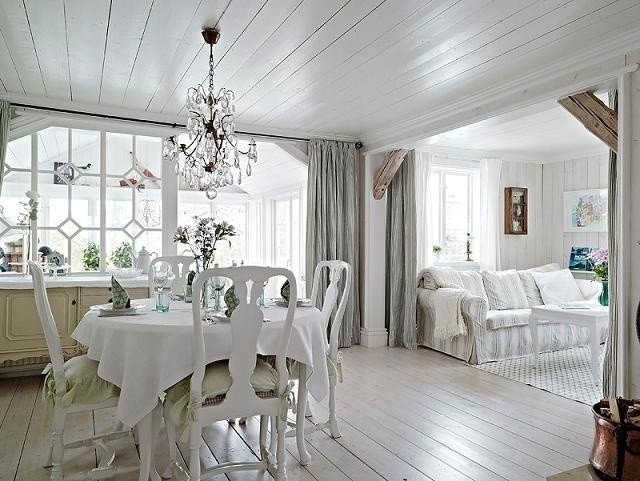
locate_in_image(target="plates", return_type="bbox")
[89,301,147,313]
[107,267,144,279]
[268,296,312,307]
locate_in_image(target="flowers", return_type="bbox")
[585,246,608,282]
[16,189,41,274]
[172,215,236,302]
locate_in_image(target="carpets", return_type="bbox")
[459,343,606,406]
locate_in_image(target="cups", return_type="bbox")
[183,284,192,303]
[256,277,270,310]
[154,290,171,313]
[211,274,226,314]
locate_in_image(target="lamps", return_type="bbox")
[162,29,258,199]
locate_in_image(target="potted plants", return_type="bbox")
[433,244,442,265]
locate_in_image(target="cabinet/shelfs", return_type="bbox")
[505,186,528,234]
[0,276,163,373]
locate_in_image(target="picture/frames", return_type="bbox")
[564,188,608,233]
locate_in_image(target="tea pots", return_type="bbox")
[130,246,159,275]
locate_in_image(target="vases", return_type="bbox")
[197,261,210,307]
[597,280,608,305]
[22,232,33,278]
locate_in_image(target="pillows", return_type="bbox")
[458,270,492,310]
[478,268,531,310]
[516,262,561,307]
[529,267,585,304]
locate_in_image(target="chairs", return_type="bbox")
[27,259,162,481]
[238,260,352,458]
[160,266,297,480]
[149,255,196,298]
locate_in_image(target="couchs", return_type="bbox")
[416,272,606,364]
